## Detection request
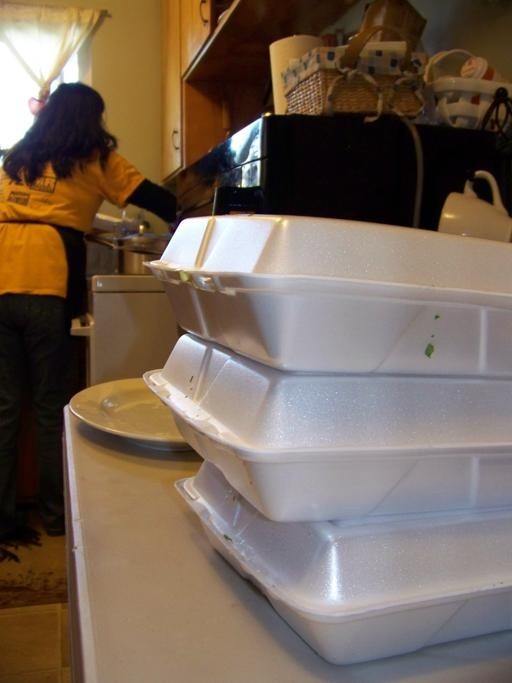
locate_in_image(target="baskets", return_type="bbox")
[281,43,437,131]
[414,46,512,133]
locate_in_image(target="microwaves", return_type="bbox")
[166,113,512,272]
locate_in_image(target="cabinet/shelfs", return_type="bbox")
[161,0,357,182]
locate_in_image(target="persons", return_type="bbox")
[0,80,178,545]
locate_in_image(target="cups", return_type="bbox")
[437,170,511,242]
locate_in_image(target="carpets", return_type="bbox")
[1,520,69,618]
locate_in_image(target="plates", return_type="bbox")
[69,377,197,452]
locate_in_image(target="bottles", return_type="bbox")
[460,56,503,81]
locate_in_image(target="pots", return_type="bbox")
[85,220,167,276]
